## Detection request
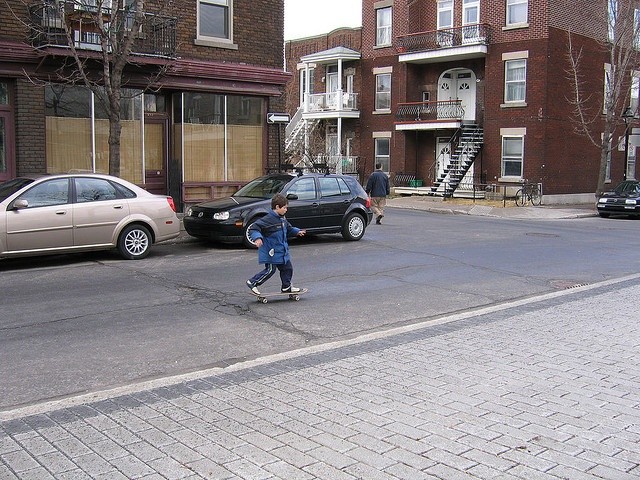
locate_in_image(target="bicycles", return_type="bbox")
[516,181,541,206]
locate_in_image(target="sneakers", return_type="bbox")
[244,279,261,297]
[281,284,300,293]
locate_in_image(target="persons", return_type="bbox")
[246,193,306,296]
[365,162,391,225]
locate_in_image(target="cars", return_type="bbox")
[0,170,180,262]
[595,180,640,219]
[185,173,375,246]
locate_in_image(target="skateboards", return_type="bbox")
[253,287,308,304]
